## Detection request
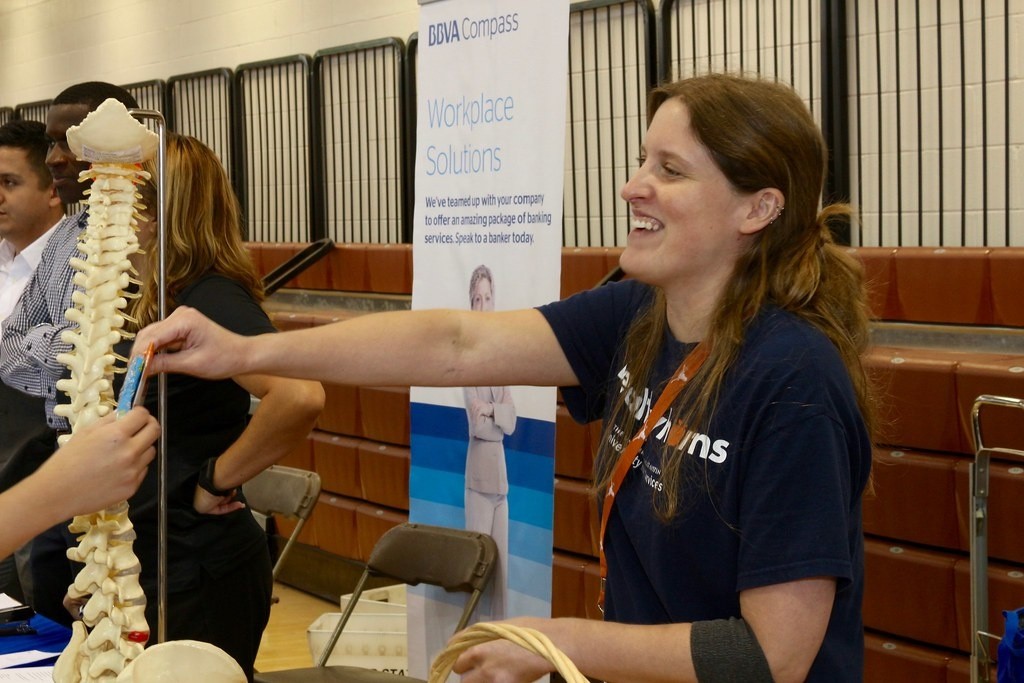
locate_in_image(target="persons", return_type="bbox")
[1,407,159,564]
[52,131,326,683]
[0,81,146,624]
[0,119,69,610]
[126,73,884,683]
[459,263,516,635]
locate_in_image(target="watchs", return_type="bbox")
[197,457,236,498]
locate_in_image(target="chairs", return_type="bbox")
[252,522,497,683]
[242,465,321,581]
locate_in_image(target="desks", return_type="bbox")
[0,613,73,683]
